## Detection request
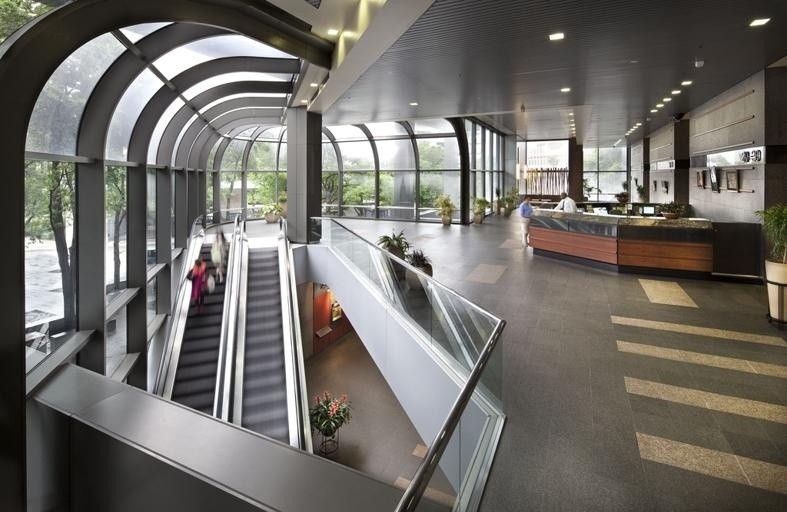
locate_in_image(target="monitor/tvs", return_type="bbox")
[593,206,608,214]
[643,206,655,215]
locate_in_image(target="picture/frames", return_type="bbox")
[696,165,740,194]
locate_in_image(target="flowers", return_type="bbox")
[309,390,355,438]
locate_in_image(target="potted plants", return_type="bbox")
[434,194,523,226]
[654,200,688,219]
[754,200,787,331]
[262,193,288,224]
[376,228,433,292]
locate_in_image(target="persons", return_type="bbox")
[520,195,532,249]
[553,192,577,215]
[190,260,207,313]
[210,227,227,284]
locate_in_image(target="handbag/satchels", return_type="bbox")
[187,269,197,282]
[205,273,216,295]
